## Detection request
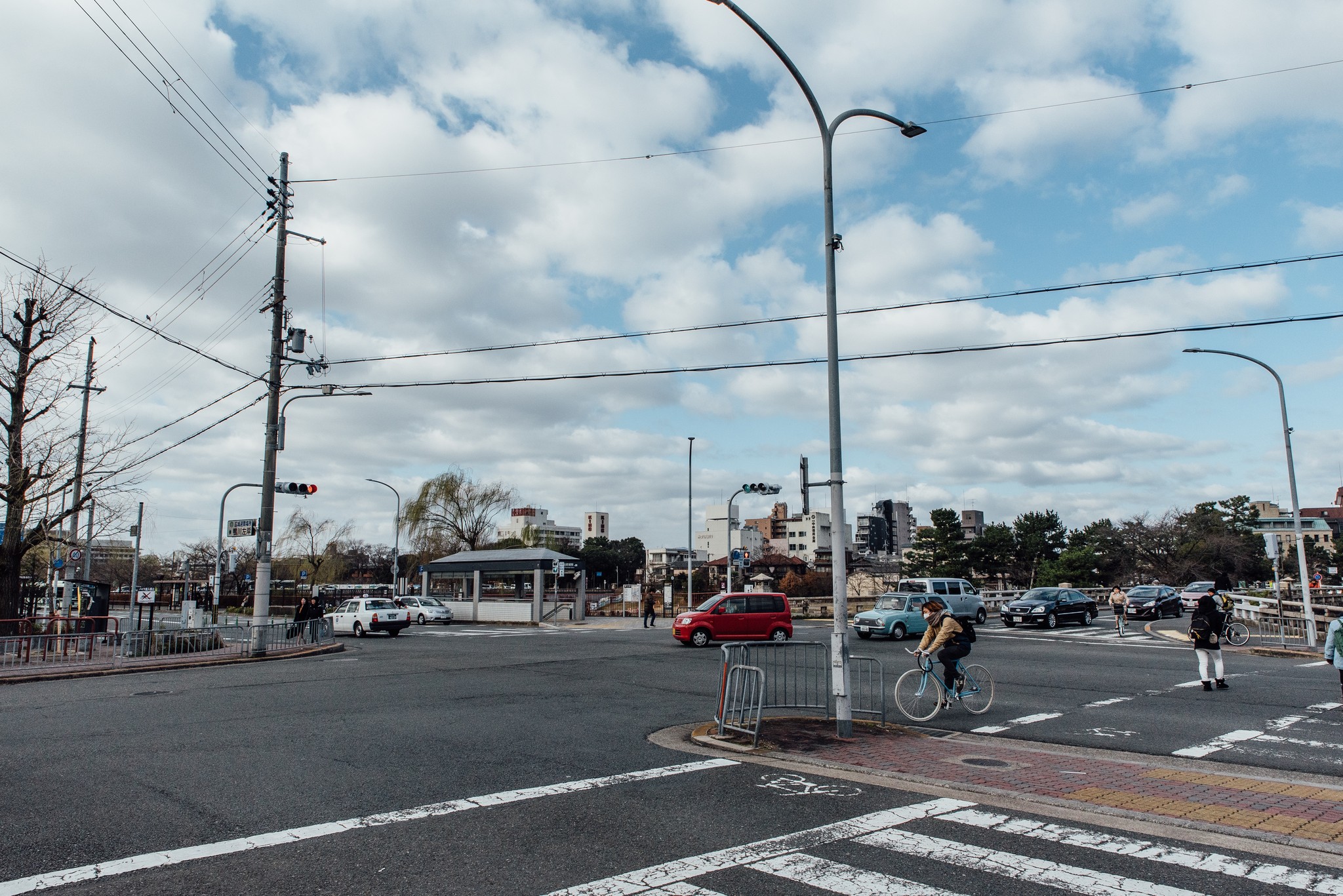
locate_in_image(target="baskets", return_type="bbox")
[1111,608,1124,615]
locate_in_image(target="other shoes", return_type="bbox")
[644,626,649,628]
[650,624,656,627]
[1222,640,1231,645]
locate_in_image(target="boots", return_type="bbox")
[1201,680,1213,691]
[1215,678,1229,690]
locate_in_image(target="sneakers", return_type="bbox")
[933,699,952,709]
[955,673,966,693]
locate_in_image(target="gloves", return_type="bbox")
[1326,659,1334,665]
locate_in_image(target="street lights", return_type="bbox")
[364,477,400,602]
[707,0,929,742]
[1182,345,1323,651]
[688,436,695,611]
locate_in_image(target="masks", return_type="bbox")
[923,611,934,619]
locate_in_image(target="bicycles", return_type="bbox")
[1111,602,1126,637]
[894,647,994,722]
[1187,612,1250,646]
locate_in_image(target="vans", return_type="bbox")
[898,578,987,625]
[114,586,142,594]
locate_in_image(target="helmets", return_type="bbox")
[394,596,400,601]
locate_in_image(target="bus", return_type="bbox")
[241,578,296,596]
[20,579,82,611]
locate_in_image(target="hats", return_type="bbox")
[312,596,319,605]
[1207,588,1216,593]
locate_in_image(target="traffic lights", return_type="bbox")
[552,560,558,573]
[1262,533,1279,559]
[743,551,750,566]
[742,483,768,493]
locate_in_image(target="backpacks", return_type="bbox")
[1334,619,1343,657]
[1191,609,1217,644]
[940,614,977,643]
[1211,593,1235,611]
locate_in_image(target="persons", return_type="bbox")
[1324,611,1343,712]
[208,592,212,610]
[243,593,250,608]
[1190,595,1229,691]
[195,592,203,609]
[1309,577,1320,594]
[1109,585,1129,630]
[644,588,657,628]
[293,597,310,647]
[309,596,323,645]
[1207,588,1232,645]
[915,600,972,708]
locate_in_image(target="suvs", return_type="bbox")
[673,592,793,647]
[397,596,453,625]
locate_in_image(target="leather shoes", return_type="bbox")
[1125,623,1128,625]
[1115,627,1119,630]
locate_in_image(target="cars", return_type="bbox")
[373,587,389,593]
[1179,581,1216,612]
[1000,587,1099,629]
[1125,586,1183,620]
[852,591,953,640]
[323,598,411,638]
[480,582,532,591]
[318,586,340,592]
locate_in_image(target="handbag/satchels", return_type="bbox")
[286,624,297,640]
